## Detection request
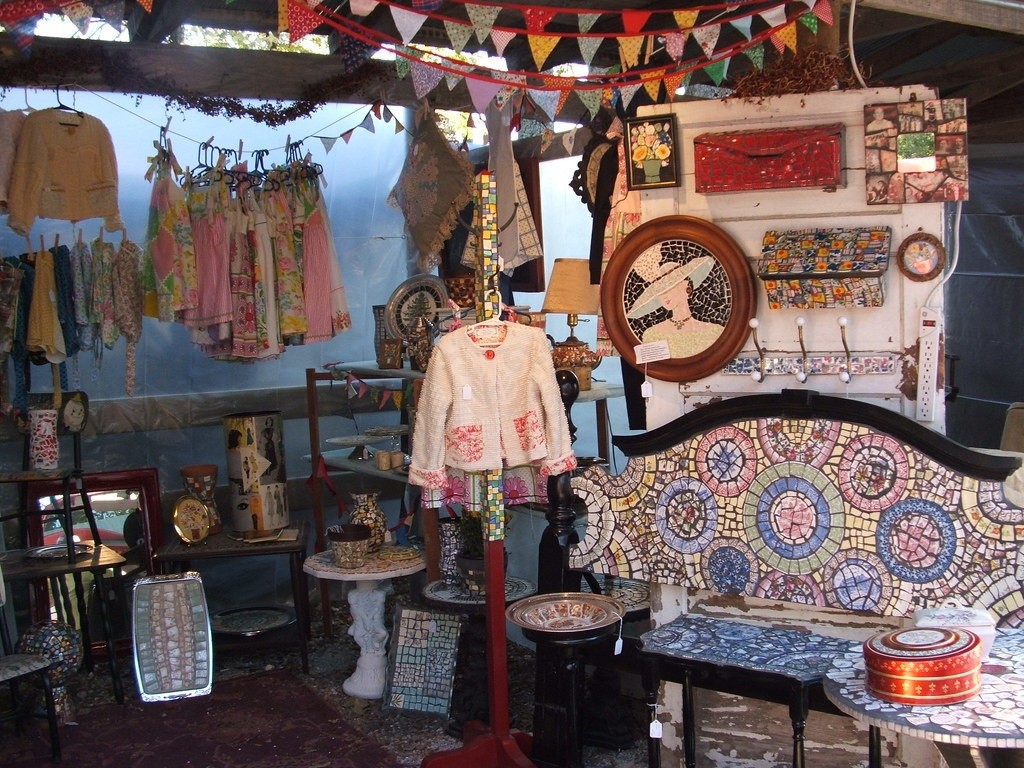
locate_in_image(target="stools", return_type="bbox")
[1,654,62,765]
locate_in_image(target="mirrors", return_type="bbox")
[21,469,160,663]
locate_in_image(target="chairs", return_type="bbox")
[0,392,101,563]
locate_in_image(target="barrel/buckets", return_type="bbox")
[328,524,371,569]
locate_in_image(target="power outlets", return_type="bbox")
[915,310,940,421]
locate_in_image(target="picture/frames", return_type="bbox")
[603,215,758,385]
[378,340,403,369]
[624,114,682,190]
[897,235,944,281]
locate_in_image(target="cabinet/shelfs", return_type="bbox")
[308,363,450,641]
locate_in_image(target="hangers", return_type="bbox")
[155,131,323,195]
[468,286,507,348]
[10,87,88,126]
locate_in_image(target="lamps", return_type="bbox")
[541,256,601,390]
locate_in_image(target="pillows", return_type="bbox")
[386,108,478,266]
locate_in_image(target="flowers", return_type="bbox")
[448,512,516,558]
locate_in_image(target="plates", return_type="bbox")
[24,545,93,558]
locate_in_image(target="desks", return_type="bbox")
[422,578,533,740]
[560,578,655,750]
[568,379,625,473]
[156,517,316,677]
[825,627,1024,767]
[0,532,129,704]
[639,617,860,767]
[302,548,427,698]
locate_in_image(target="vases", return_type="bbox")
[454,547,510,593]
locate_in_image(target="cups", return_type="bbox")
[377,450,403,471]
